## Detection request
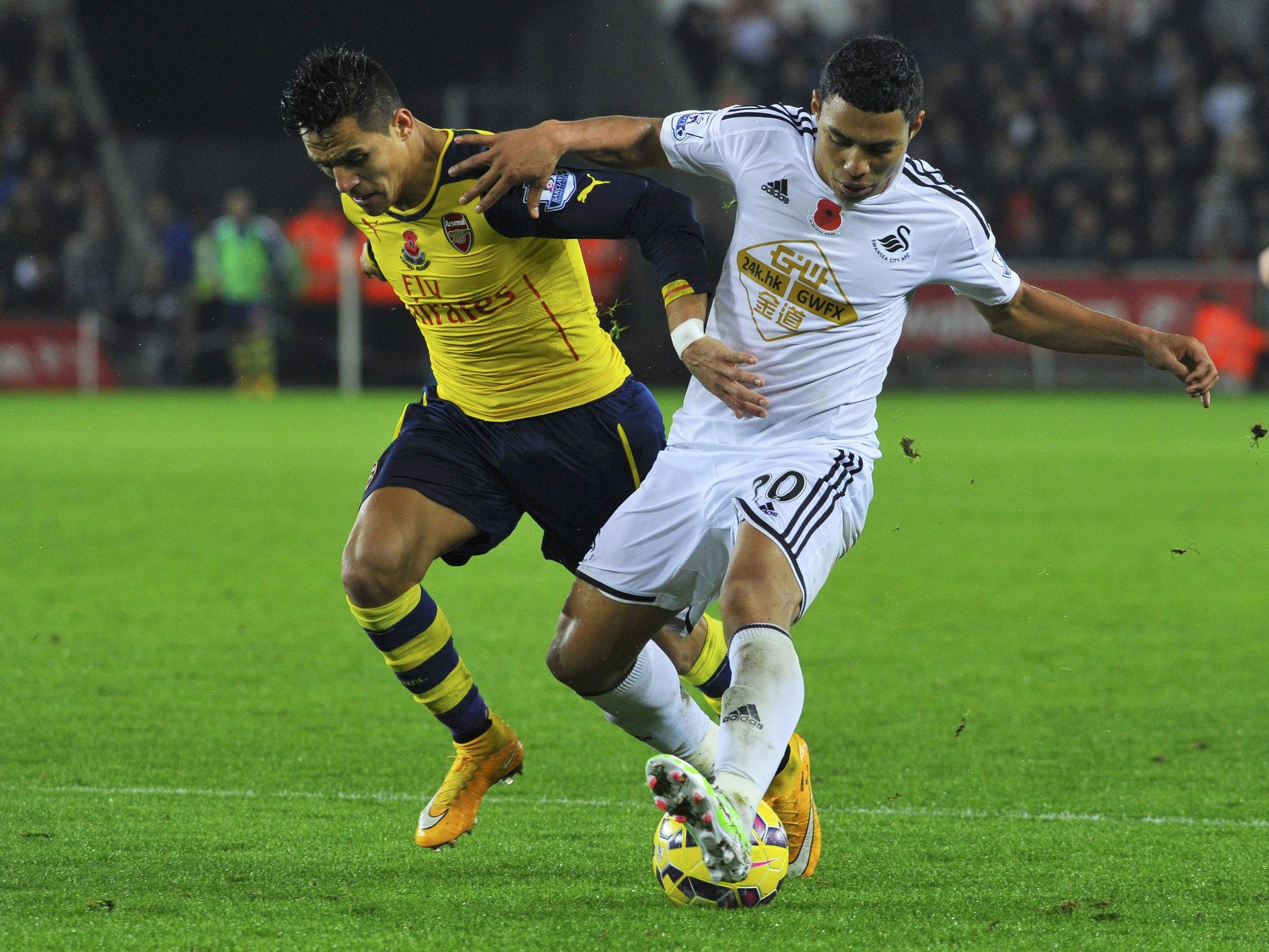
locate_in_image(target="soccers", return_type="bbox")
[651,802,790,913]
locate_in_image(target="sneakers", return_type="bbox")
[414,707,525,849]
[645,754,753,885]
[763,732,822,878]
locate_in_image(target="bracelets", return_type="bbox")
[671,318,705,360]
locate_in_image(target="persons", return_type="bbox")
[279,48,824,876]
[195,184,301,391]
[0,0,117,320]
[144,189,199,296]
[677,0,1269,265]
[448,36,1220,883]
